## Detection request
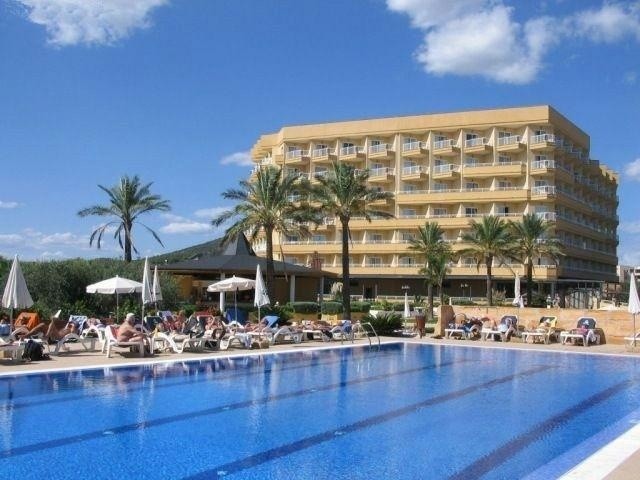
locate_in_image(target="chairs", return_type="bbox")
[0,305,370,359]
[444,318,481,341]
[480,315,523,343]
[624,332,640,345]
[559,317,601,347]
[521,316,564,345]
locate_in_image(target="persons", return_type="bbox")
[415,308,427,339]
[570,323,590,335]
[0,313,361,358]
[526,321,549,333]
[546,296,560,310]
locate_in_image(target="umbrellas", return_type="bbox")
[253,263,270,331]
[0,254,35,325]
[85,275,143,324]
[151,265,163,316]
[139,257,152,333]
[207,274,256,327]
[629,272,640,346]
[513,270,524,330]
